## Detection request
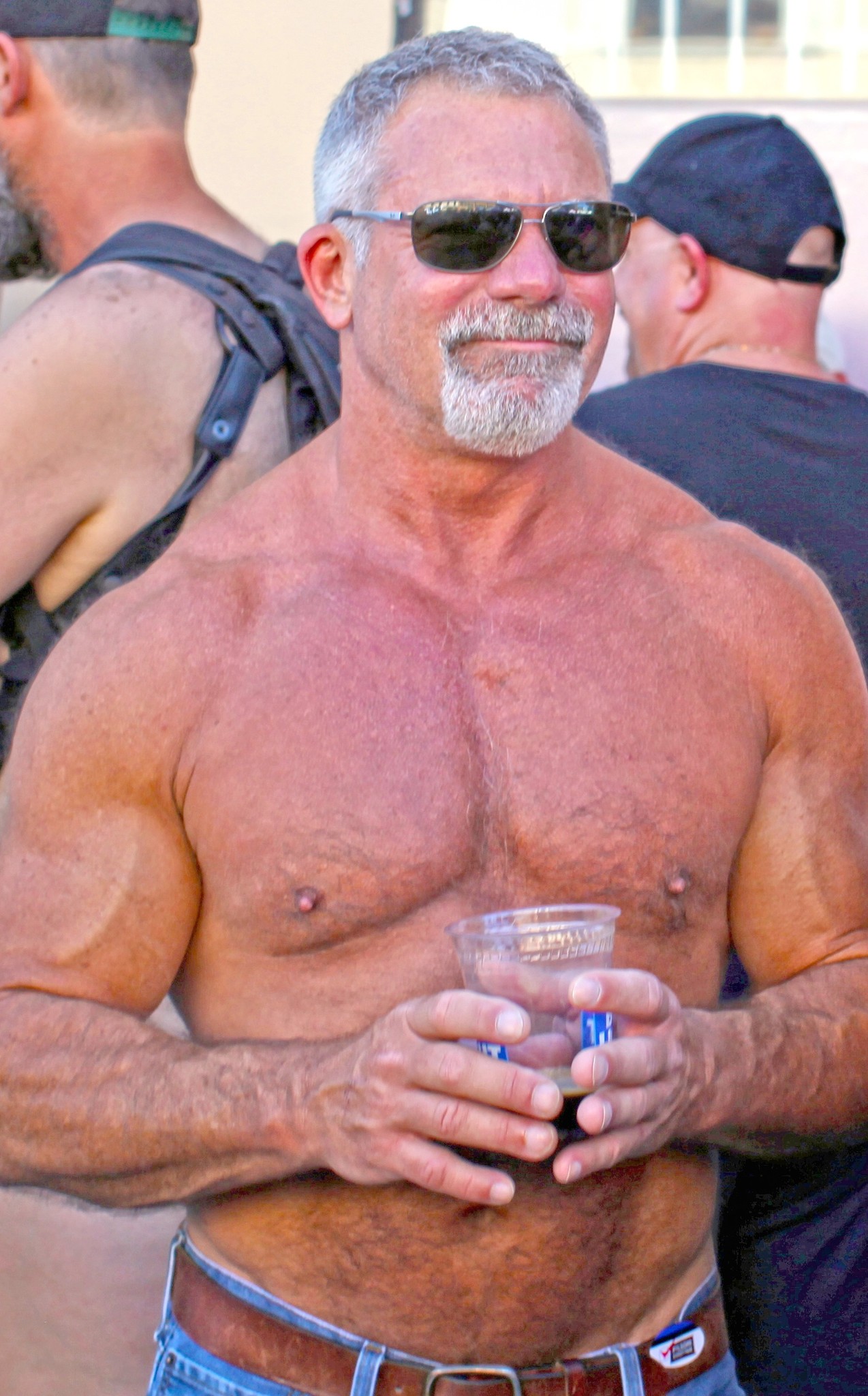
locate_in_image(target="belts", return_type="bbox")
[173,1238,732,1395]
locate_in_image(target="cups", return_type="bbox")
[445,902,623,1169]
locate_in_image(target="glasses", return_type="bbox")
[327,198,640,278]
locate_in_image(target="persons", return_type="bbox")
[566,112,868,1396]
[1,0,342,767]
[0,29,868,1396]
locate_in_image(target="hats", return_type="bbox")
[612,109,847,287]
